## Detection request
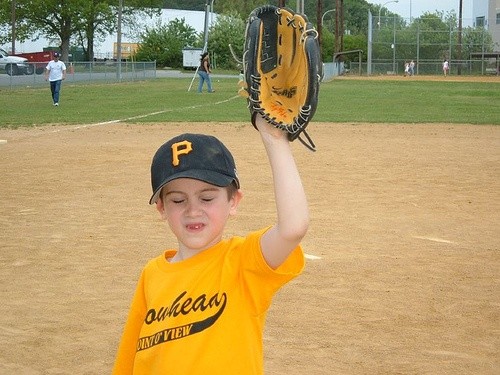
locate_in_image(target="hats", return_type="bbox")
[54,53,59,57]
[149,133,239,205]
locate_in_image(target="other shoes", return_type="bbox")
[209,90,215,93]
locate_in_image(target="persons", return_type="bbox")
[111,77,310,375]
[198,52,215,93]
[44,53,67,106]
[404,59,450,77]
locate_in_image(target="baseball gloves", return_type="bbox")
[227,4,338,152]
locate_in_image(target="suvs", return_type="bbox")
[0,49,29,77]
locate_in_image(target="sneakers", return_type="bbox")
[55,103,58,106]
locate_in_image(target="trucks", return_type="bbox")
[15,51,56,74]
